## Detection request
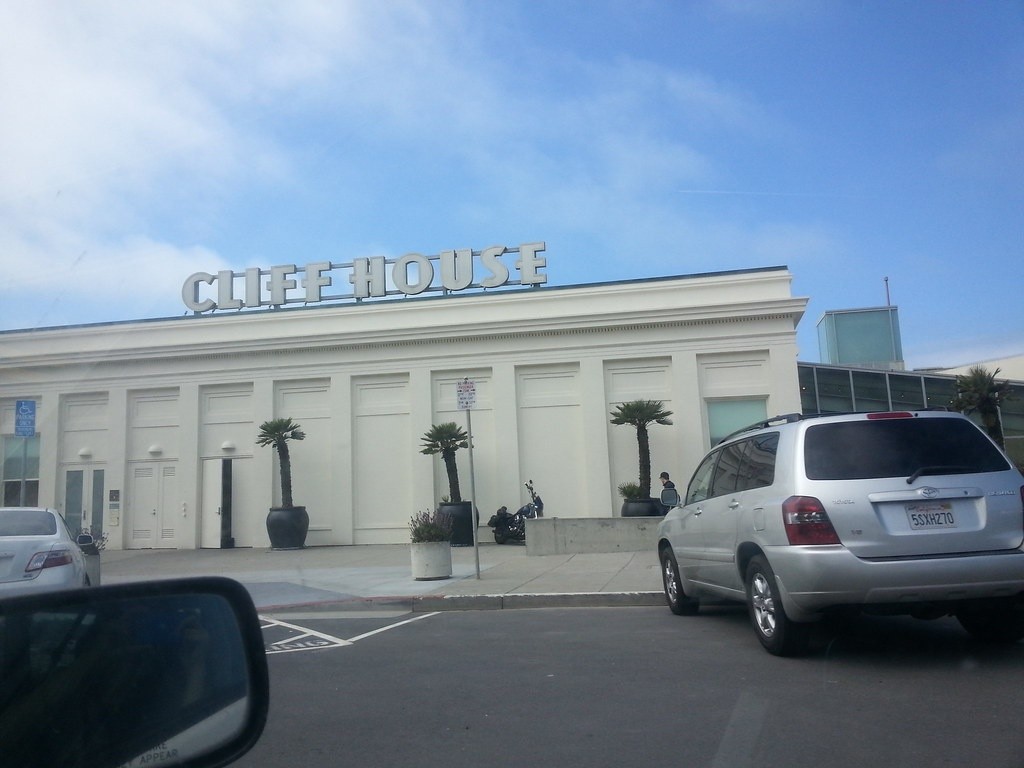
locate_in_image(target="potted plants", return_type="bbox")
[417,420,481,547]
[610,398,675,515]
[256,415,311,551]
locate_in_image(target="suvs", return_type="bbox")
[654,412,1024,658]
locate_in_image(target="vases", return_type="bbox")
[409,541,453,579]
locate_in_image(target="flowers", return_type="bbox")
[405,507,455,541]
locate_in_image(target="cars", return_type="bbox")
[0,507,93,600]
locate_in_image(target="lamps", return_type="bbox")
[78,447,92,456]
[222,442,235,450]
[147,445,162,454]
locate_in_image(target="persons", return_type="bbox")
[659,472,675,515]
